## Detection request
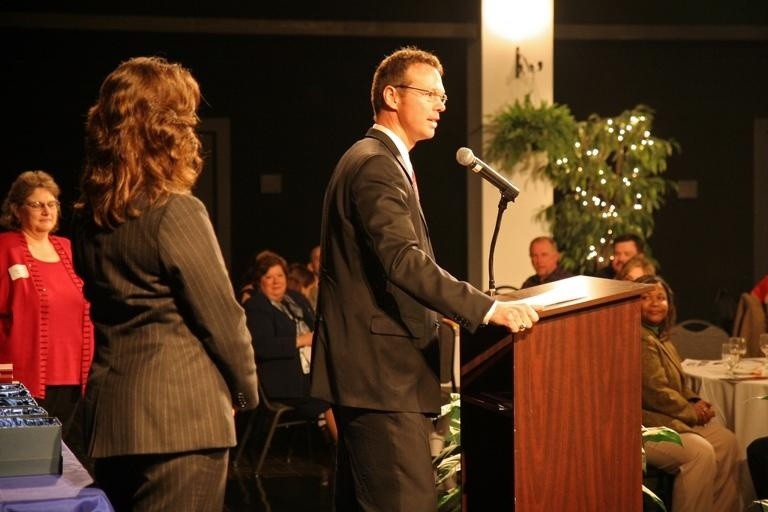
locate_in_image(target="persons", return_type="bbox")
[303,245,321,313]
[522,235,576,288]
[597,233,644,279]
[308,49,545,512]
[617,256,656,284]
[0,169,95,454]
[72,59,259,511]
[241,253,340,465]
[630,272,741,512]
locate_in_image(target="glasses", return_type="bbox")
[22,201,57,209]
[393,82,449,105]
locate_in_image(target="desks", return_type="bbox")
[0,442,114,512]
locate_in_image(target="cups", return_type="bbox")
[722,344,740,379]
[729,337,746,369]
[759,333,768,366]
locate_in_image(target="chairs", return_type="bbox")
[232,382,308,478]
[440,318,460,512]
[669,321,729,359]
[733,294,765,357]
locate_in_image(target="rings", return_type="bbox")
[518,322,525,328]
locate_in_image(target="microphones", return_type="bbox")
[457,148,520,211]
